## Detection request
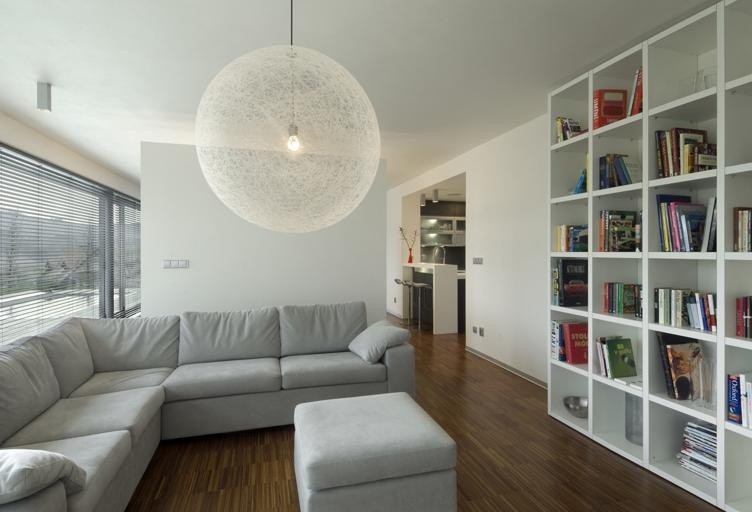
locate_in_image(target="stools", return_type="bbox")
[292,390,459,512]
[394,277,432,331]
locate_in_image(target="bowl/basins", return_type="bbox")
[563,395,589,417]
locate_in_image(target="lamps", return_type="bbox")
[188,1,385,236]
[419,190,439,206]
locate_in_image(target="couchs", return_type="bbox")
[2,300,422,511]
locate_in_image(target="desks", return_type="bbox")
[402,262,458,334]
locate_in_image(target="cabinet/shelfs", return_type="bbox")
[421,216,465,247]
[547,1,752,512]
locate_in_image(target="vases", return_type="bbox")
[408,247,413,262]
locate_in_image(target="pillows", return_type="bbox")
[2,446,87,501]
[347,319,410,365]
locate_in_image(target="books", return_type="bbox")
[572,169,586,194]
[555,116,581,143]
[674,421,718,483]
[631,65,642,116]
[626,68,639,116]
[652,126,717,179]
[593,88,628,130]
[598,153,642,190]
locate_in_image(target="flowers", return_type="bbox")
[398,225,418,248]
